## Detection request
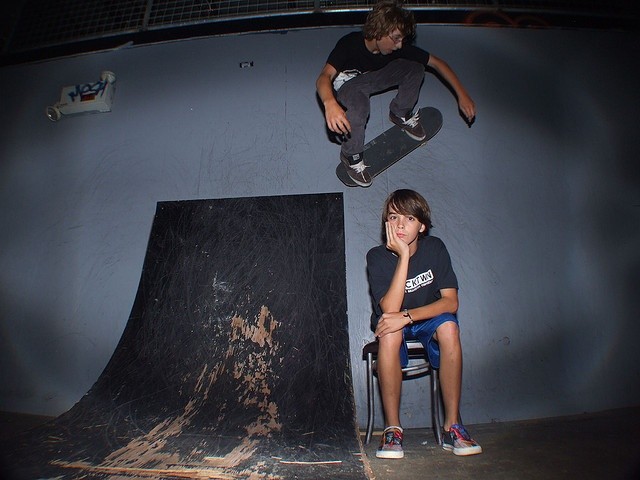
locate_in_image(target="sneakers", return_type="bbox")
[389,107,427,141]
[340,151,373,186]
[443,423,482,455]
[376,426,404,458]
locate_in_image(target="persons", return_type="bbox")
[316,0,477,186]
[366,189,482,458]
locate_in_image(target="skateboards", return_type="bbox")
[335,104,444,190]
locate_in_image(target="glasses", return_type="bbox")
[388,33,405,43]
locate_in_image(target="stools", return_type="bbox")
[363,339,444,445]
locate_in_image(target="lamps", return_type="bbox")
[45,106,61,122]
[100,70,117,84]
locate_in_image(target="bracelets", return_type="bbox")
[403,309,414,324]
